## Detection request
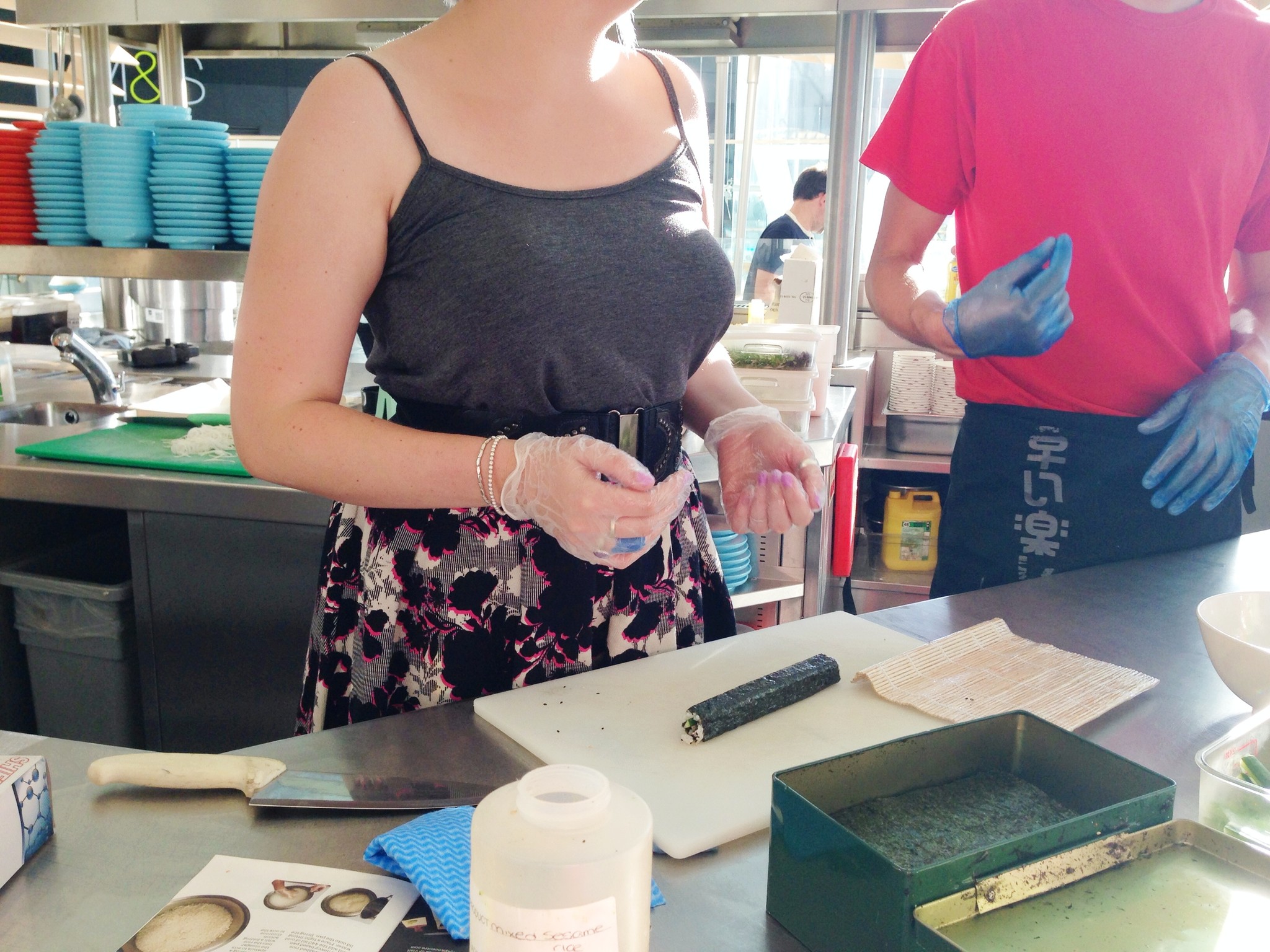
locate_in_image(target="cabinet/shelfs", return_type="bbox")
[834,426,952,616]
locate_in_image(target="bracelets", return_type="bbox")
[475,435,506,516]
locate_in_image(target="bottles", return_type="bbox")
[856,274,874,312]
[469,763,654,951]
[746,299,764,323]
[944,250,962,304]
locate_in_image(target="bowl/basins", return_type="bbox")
[1194,706,1270,851]
[889,350,967,417]
[862,468,943,532]
[320,887,378,917]
[264,886,313,910]
[117,895,251,952]
[1196,592,1270,710]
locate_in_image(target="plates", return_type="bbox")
[0,103,279,249]
[703,513,752,591]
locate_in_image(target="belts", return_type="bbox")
[396,400,683,481]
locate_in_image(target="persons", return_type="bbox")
[229,1,829,735]
[859,0,1270,597]
[744,169,827,312]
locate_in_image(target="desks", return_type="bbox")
[0,528,1270,952]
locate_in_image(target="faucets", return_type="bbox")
[51,325,129,405]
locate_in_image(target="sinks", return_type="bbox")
[0,399,133,426]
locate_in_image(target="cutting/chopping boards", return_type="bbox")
[472,610,960,860]
[14,421,253,477]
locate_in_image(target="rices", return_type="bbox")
[135,903,233,952]
[329,892,371,912]
[269,888,308,907]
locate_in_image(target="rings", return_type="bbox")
[609,518,618,537]
[798,458,820,470]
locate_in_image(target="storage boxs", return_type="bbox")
[719,323,841,442]
[882,390,963,454]
[766,706,1270,952]
[0,756,54,890]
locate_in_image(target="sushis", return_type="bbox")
[681,654,841,744]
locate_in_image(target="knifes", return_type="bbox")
[88,751,501,809]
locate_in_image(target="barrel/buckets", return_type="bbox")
[881,490,941,572]
